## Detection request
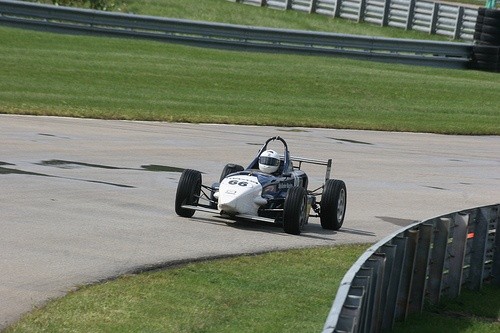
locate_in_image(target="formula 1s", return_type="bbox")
[175,135,350,235]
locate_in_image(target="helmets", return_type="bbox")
[259,150,280,174]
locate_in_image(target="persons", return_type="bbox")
[259,150,282,176]
